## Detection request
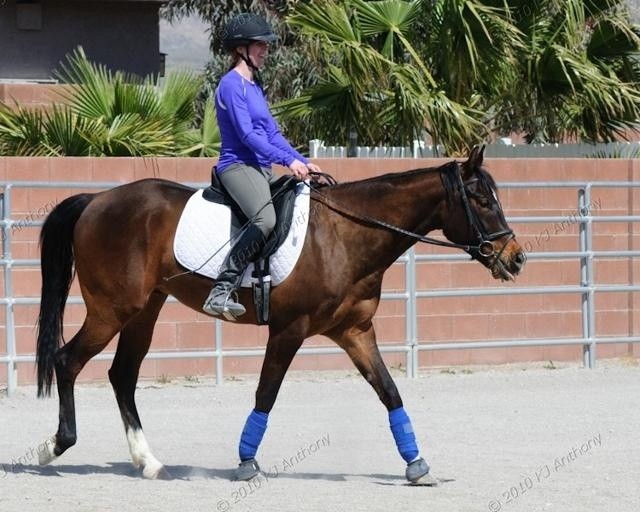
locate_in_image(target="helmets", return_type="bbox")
[223,13,282,49]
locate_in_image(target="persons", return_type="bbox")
[202,13,322,316]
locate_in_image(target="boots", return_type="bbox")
[203,224,266,317]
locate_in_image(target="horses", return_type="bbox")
[13,144,527,486]
